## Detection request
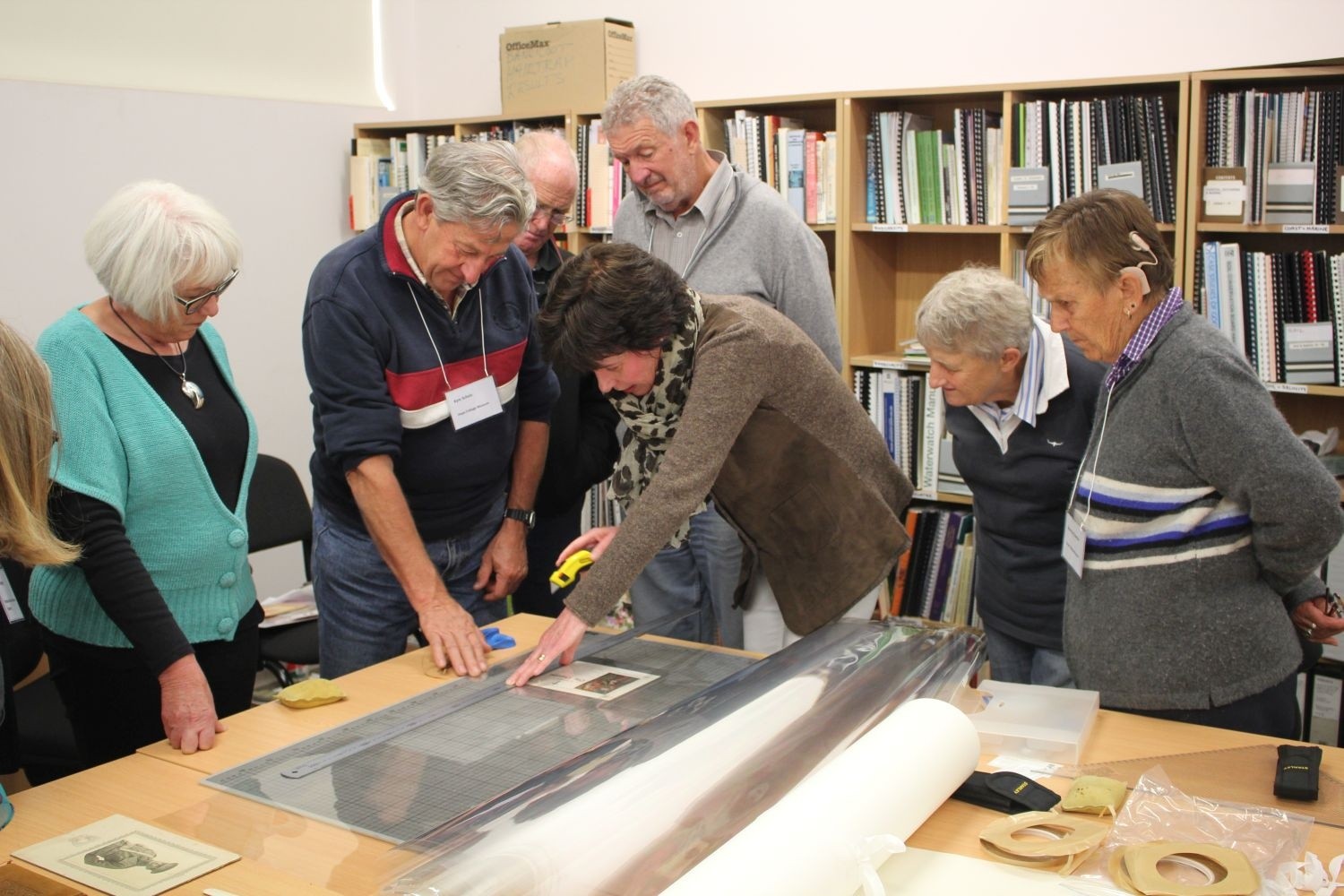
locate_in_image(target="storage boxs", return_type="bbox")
[498,16,638,115]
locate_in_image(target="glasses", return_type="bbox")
[169,268,240,315]
[532,204,573,227]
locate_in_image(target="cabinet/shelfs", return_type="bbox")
[843,68,1188,631]
[573,91,843,366]
[1185,68,1343,753]
[347,110,573,255]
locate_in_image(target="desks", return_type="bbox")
[0,613,1344,896]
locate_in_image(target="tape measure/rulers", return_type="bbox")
[277,604,705,780]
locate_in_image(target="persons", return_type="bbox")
[1027,185,1342,742]
[502,240,916,690]
[600,73,843,657]
[506,129,622,619]
[31,175,270,769]
[299,139,565,680]
[913,258,1112,692]
[0,317,87,787]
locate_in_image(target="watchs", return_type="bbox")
[503,507,536,529]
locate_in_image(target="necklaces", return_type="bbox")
[108,294,205,410]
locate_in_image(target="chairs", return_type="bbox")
[245,452,323,686]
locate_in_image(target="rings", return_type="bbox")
[1308,622,1317,630]
[1302,628,1313,638]
[537,653,546,661]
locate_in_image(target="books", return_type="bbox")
[1292,656,1314,743]
[718,81,1344,226]
[1322,536,1344,662]
[962,678,1101,767]
[347,115,633,233]
[1310,659,1344,749]
[849,338,991,689]
[1191,236,1344,393]
